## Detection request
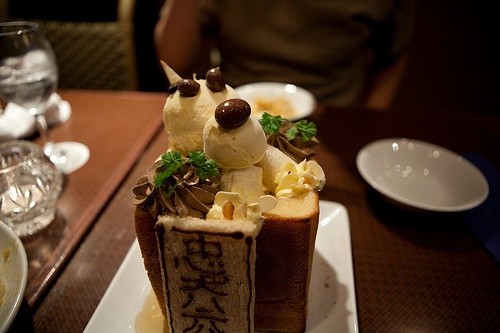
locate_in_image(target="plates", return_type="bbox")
[82,200,359,333]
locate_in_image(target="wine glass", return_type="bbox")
[0,21,88,175]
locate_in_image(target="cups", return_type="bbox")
[0,140,64,236]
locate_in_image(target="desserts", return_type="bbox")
[131,60,326,333]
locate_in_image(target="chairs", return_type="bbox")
[0,0,138,92]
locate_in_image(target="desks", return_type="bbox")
[34,107,500,333]
[0,88,169,312]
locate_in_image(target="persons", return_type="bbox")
[152,0,429,109]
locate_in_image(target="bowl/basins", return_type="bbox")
[356,138,489,213]
[0,222,28,333]
[234,81,316,120]
